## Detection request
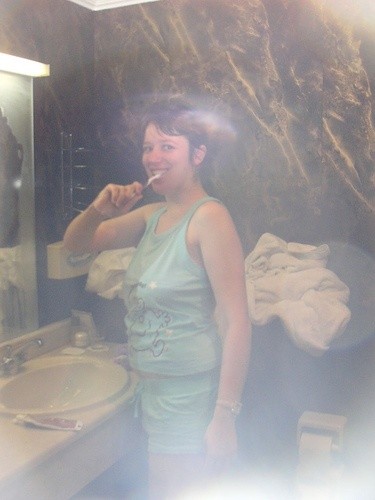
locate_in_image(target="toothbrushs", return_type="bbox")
[123,171,165,202]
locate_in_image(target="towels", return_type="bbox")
[243,232,352,353]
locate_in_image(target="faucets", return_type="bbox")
[0,338,44,376]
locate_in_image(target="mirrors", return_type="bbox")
[0,71,40,339]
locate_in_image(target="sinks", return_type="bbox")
[0,353,130,416]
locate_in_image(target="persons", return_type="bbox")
[61,104,252,500]
